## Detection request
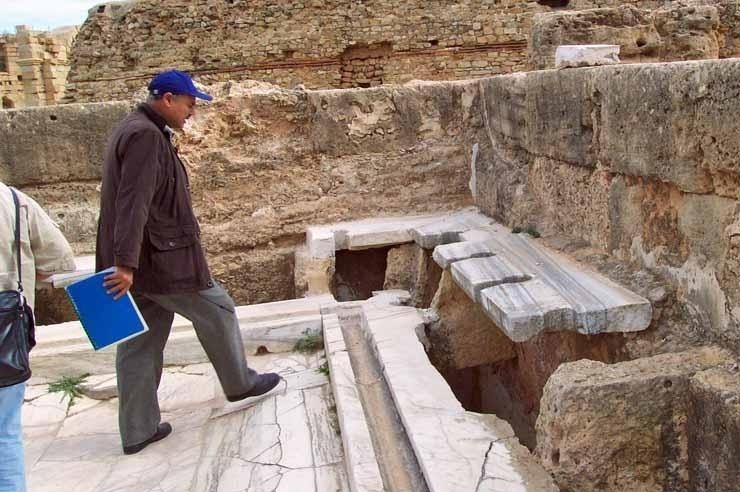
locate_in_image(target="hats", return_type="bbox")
[148,70,213,101]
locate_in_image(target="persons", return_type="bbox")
[95,70,283,457]
[0,181,81,490]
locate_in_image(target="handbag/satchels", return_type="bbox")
[0,284,36,388]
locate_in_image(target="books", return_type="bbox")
[64,267,151,353]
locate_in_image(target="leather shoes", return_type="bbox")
[124,422,172,454]
[227,373,280,402]
